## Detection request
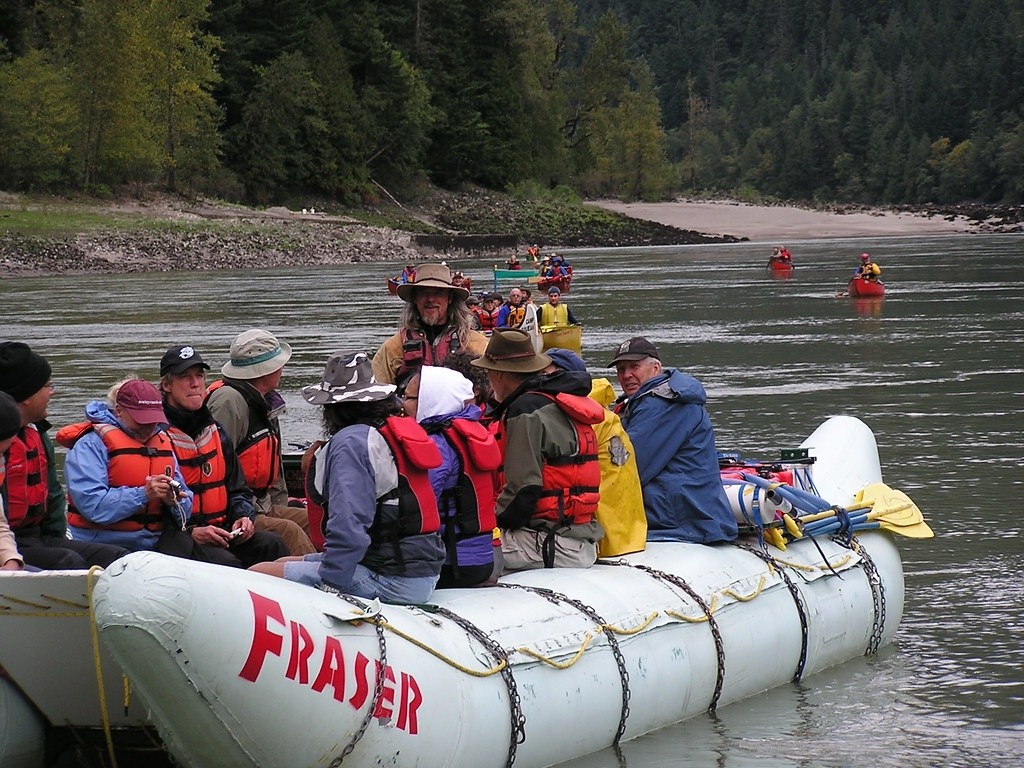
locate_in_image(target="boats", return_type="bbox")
[0,415,905,768]
[537,267,573,293]
[769,258,794,270]
[494,255,541,278]
[847,273,885,299]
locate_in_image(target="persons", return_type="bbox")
[204,330,317,554]
[471,327,605,570]
[544,347,647,556]
[157,345,290,568]
[606,337,739,545]
[528,245,540,257]
[443,349,499,434]
[854,253,881,282]
[0,397,25,571]
[0,341,131,570]
[768,245,793,264]
[401,263,416,284]
[372,265,495,400]
[451,271,578,332]
[54,381,194,560]
[247,352,445,603]
[402,365,504,588]
[539,253,572,280]
[505,255,520,270]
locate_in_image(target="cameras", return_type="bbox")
[166,480,182,496]
[224,528,243,541]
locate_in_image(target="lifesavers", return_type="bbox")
[720,467,756,480]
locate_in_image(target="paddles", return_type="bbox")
[761,478,935,551]
[527,275,569,283]
[494,264,498,293]
[839,266,870,296]
[766,264,769,270]
[530,248,538,261]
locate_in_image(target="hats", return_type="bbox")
[0,342,50,403]
[489,292,503,302]
[471,328,552,371]
[517,285,531,298]
[395,265,469,303]
[543,347,586,372]
[547,287,562,295]
[302,351,397,405]
[221,329,292,379]
[861,253,869,261]
[159,344,211,377]
[466,296,480,304]
[606,338,660,369]
[452,271,464,278]
[483,294,493,304]
[116,380,169,425]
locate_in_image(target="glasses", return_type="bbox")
[400,395,418,403]
[42,385,55,391]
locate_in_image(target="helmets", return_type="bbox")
[541,253,560,266]
